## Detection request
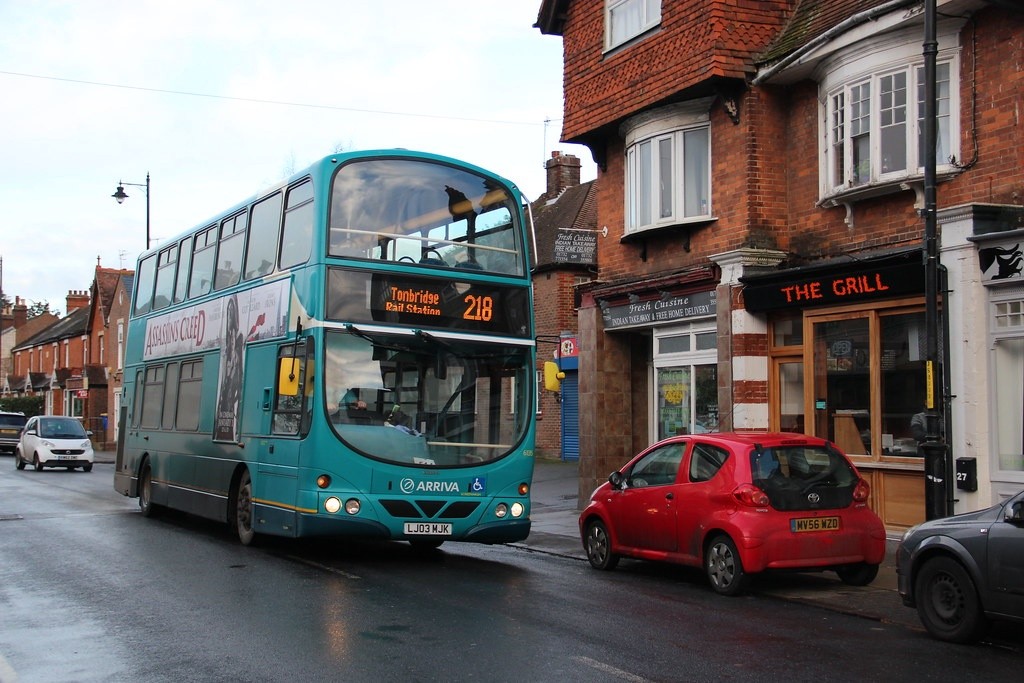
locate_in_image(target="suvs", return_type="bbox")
[0,410,27,454]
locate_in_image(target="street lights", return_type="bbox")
[113,171,153,249]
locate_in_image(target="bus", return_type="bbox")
[114,148,567,560]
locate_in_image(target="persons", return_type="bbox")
[42,421,51,436]
[912,400,945,455]
[214,294,244,441]
[308,363,367,417]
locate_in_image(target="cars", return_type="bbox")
[895,487,1024,643]
[577,432,888,594]
[15,415,95,472]
